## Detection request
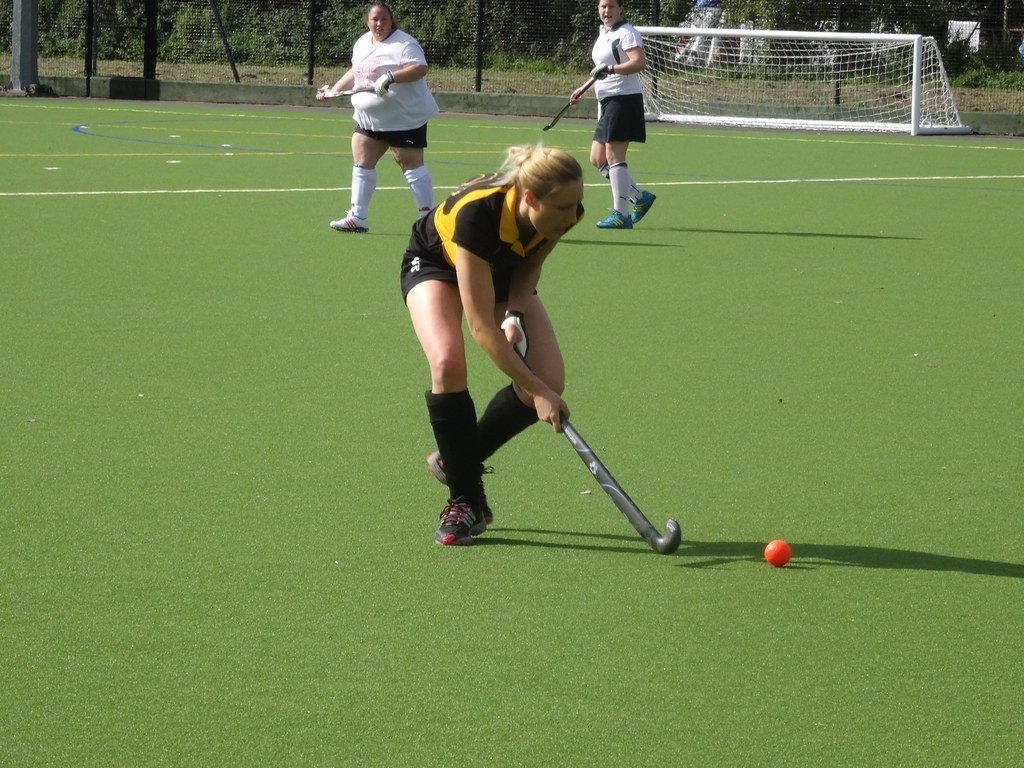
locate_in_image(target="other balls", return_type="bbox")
[764,540,791,567]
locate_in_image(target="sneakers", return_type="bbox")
[632,189,656,224]
[428,449,495,524]
[596,210,633,229]
[329,211,369,233]
[434,495,487,547]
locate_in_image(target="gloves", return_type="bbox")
[373,70,394,97]
[590,64,615,81]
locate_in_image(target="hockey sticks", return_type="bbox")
[316,84,389,98]
[512,342,682,555]
[542,75,598,131]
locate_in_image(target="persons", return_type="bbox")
[399,141,585,547]
[315,3,440,233]
[570,0,657,228]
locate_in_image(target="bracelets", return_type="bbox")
[610,65,615,74]
